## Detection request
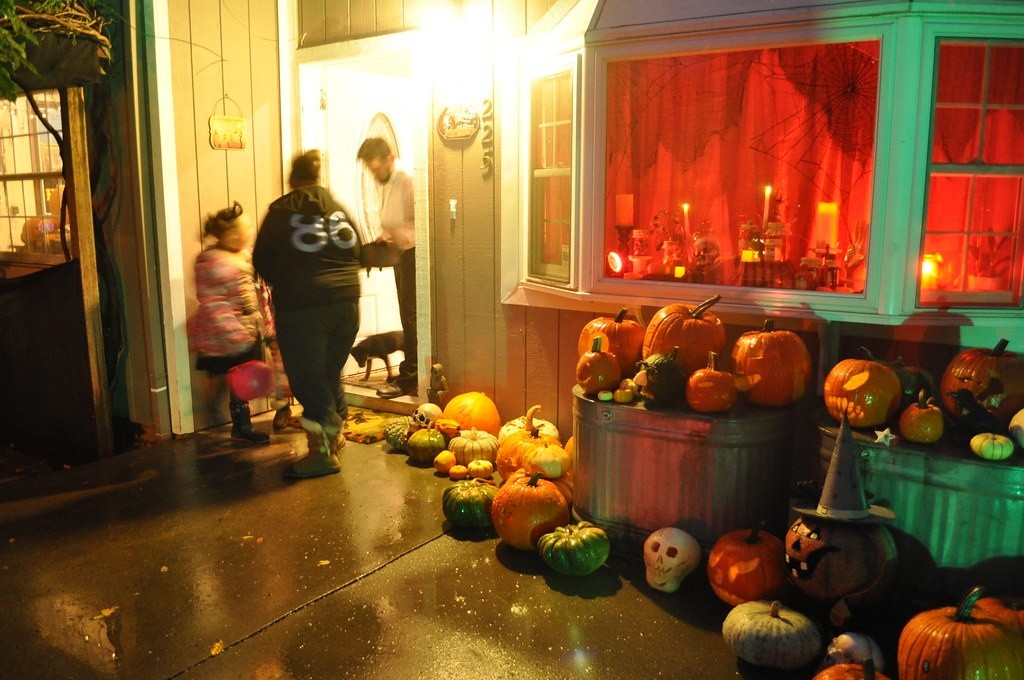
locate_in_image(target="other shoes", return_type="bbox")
[376,379,417,397]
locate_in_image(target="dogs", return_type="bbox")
[349,330,406,383]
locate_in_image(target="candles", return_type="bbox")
[684,202,691,248]
[763,185,771,229]
[817,201,839,246]
[613,195,634,226]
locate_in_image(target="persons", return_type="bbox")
[357,137,417,397]
[252,272,296,428]
[254,149,363,476]
[194,201,270,446]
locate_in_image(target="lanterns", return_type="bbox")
[825,359,903,428]
[686,369,735,413]
[497,430,564,481]
[706,526,785,607]
[490,472,570,550]
[785,515,896,602]
[574,351,621,393]
[730,327,811,405]
[941,338,1024,431]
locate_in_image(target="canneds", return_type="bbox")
[798,257,842,291]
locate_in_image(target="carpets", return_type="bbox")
[286,405,409,447]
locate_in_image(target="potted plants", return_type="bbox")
[965,227,1011,292]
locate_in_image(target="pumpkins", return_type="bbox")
[897,586,1024,680]
[641,295,724,376]
[970,407,1024,461]
[900,389,944,443]
[809,658,894,680]
[384,392,608,573]
[722,599,820,667]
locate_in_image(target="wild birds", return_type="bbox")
[946,389,1018,444]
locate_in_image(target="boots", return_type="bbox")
[271,398,292,429]
[228,402,270,443]
[282,416,341,478]
[336,434,345,449]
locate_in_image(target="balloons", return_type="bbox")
[229,361,271,400]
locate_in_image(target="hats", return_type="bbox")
[790,411,896,525]
